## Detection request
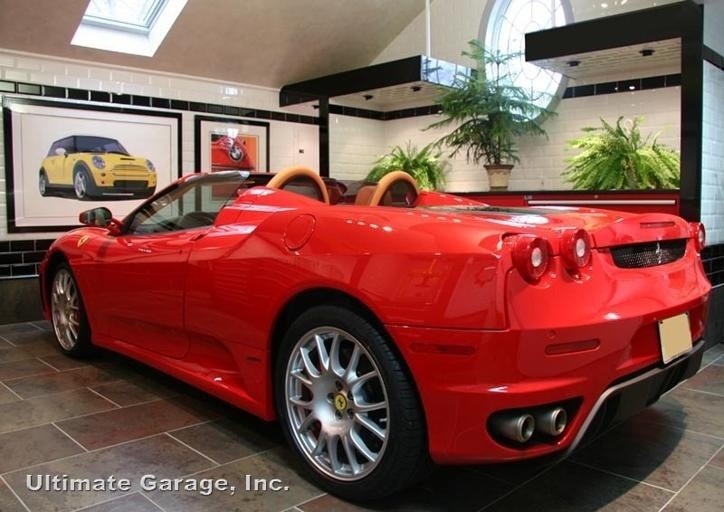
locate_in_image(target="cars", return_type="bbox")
[39,136,158,201]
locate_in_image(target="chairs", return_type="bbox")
[367,170,421,207]
[265,166,330,206]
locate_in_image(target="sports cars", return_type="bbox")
[38,168,712,506]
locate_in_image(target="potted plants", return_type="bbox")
[418,49,559,188]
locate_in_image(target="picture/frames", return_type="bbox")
[2,95,183,234]
[194,115,270,210]
